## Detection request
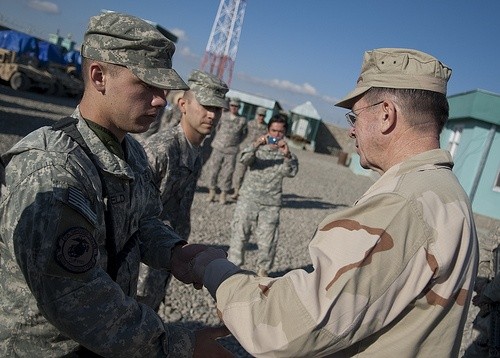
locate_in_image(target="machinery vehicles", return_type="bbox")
[0,47,83,95]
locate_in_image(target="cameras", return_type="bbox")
[269,137,278,144]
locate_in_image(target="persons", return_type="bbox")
[0,10,238,358]
[228,115,299,277]
[229,107,269,201]
[205,98,247,204]
[460,224,500,358]
[140,90,182,138]
[186,47,479,358]
[136,69,229,312]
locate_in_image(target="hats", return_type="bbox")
[255,106,266,115]
[184,70,231,108]
[344,101,387,128]
[334,48,453,107]
[81,13,190,90]
[229,97,241,107]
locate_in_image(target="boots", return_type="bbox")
[206,189,217,202]
[230,189,240,199]
[219,192,227,205]
[257,268,267,277]
